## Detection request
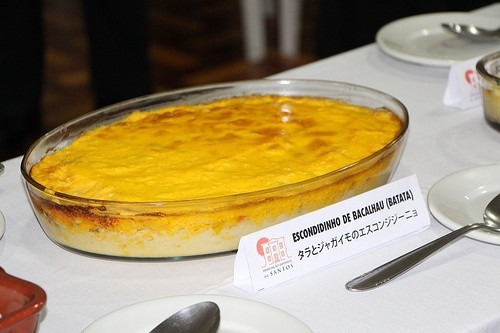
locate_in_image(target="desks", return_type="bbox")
[0,4,500,333]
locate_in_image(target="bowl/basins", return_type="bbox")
[0,271,48,333]
[474,50,500,131]
[19,77,409,263]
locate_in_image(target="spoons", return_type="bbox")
[345,194,500,292]
[438,21,500,43]
[148,301,220,333]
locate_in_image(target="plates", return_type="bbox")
[376,11,500,68]
[81,293,314,333]
[427,164,500,245]
